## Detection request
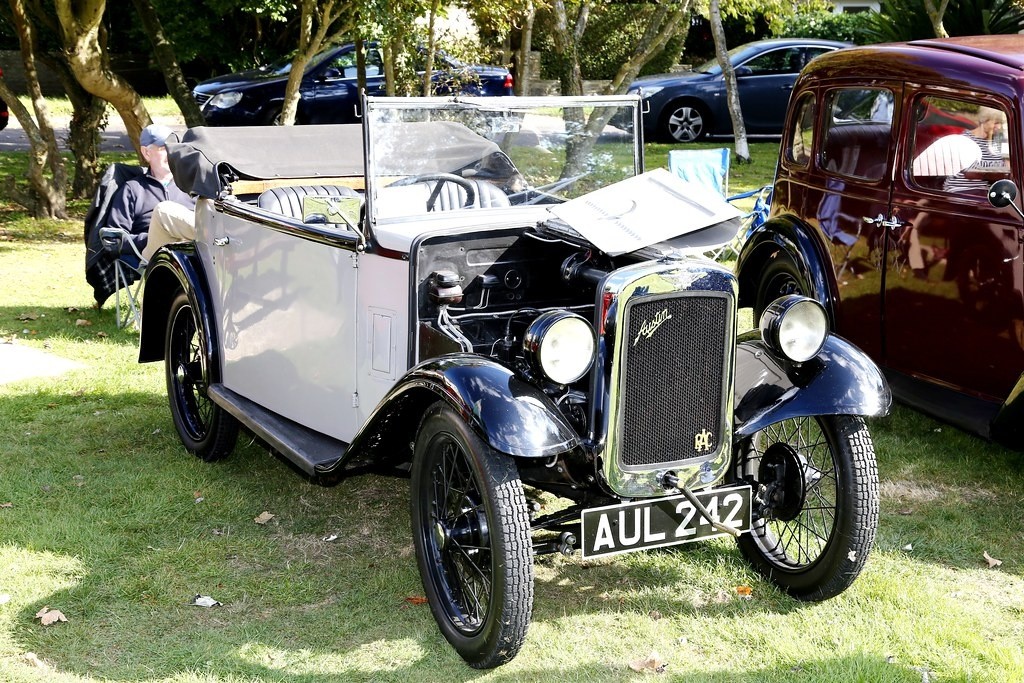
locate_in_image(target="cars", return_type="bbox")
[735,32,1024,463]
[136,88,894,669]
[618,37,882,144]
[191,40,514,129]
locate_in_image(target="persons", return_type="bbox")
[106,125,196,261]
[942,107,1009,197]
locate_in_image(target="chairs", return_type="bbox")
[668,148,772,263]
[373,180,509,220]
[105,161,150,330]
[257,184,366,233]
[787,54,800,73]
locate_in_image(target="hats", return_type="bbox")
[139,124,173,146]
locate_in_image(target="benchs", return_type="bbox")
[824,122,972,191]
